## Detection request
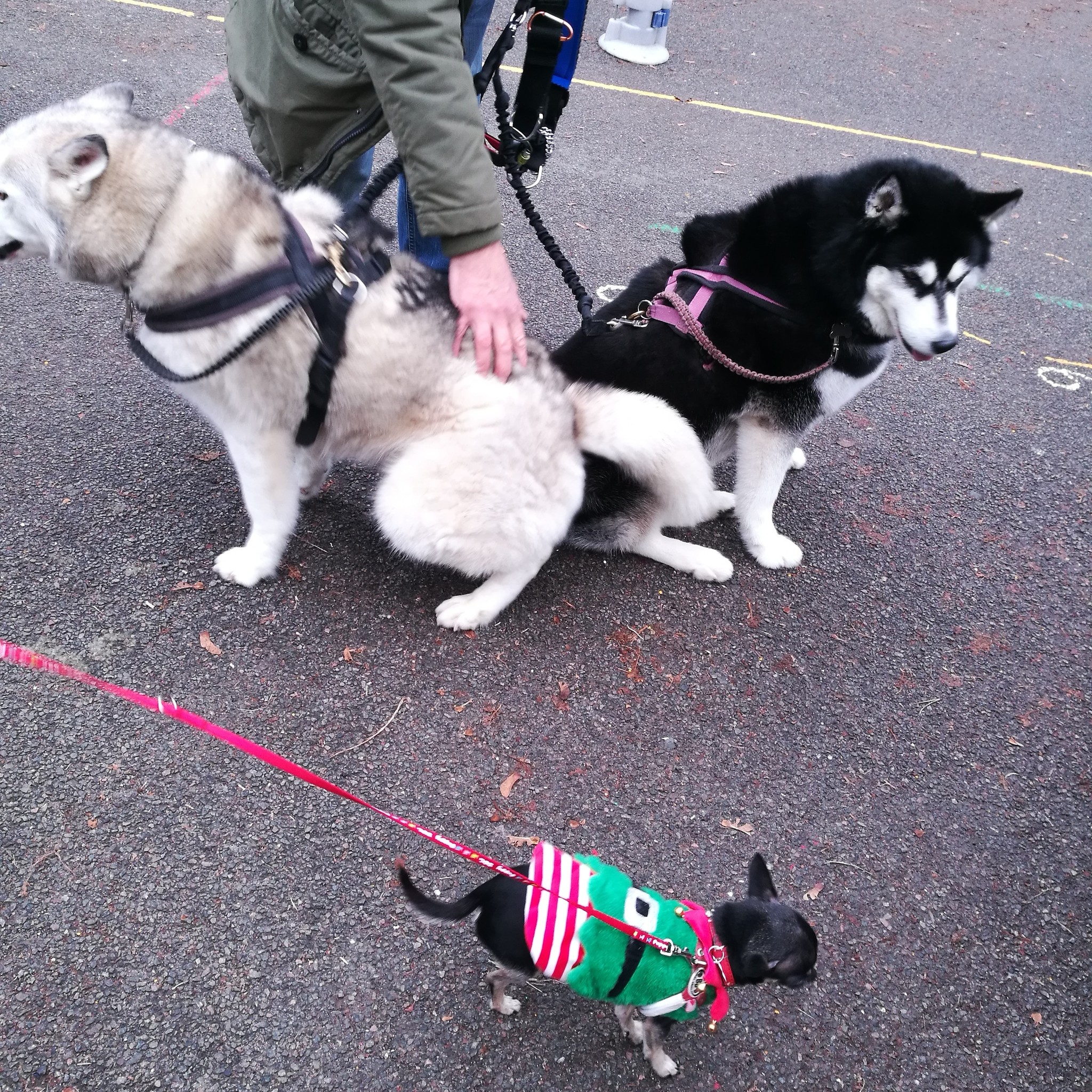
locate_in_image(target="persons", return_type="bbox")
[221,1,537,385]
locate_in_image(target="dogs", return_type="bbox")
[393,848,819,1078]
[537,159,1025,587]
[2,83,723,633]
